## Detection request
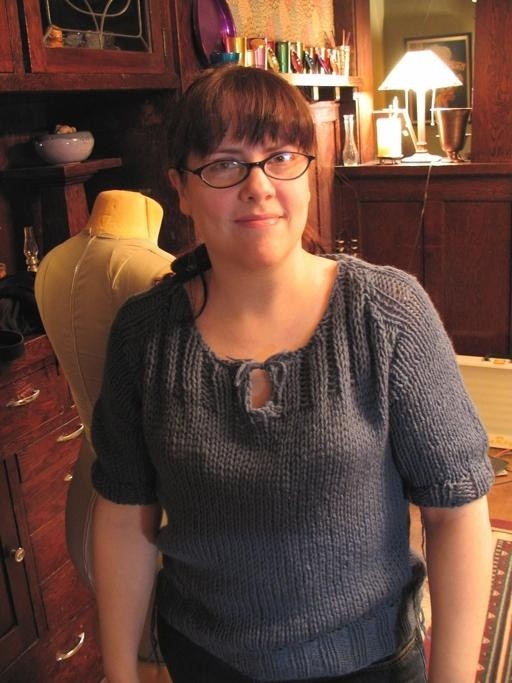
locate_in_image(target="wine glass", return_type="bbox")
[431,106,470,164]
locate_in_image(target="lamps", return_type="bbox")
[376,50,463,162]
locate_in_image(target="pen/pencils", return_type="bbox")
[323,28,351,47]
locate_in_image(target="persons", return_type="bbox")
[90,66,495,683]
[34,189,181,664]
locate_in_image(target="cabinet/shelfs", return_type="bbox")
[1,0,196,91]
[300,101,343,257]
[0,247,197,682]
[336,164,511,359]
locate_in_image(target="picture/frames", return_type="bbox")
[402,34,471,126]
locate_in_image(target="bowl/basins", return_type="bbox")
[33,130,95,164]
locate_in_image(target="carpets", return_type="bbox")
[413,440,512,683]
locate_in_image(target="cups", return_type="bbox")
[340,113,359,167]
[211,37,351,75]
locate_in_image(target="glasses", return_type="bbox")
[171,150,317,192]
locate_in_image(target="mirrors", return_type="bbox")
[369,1,475,163]
